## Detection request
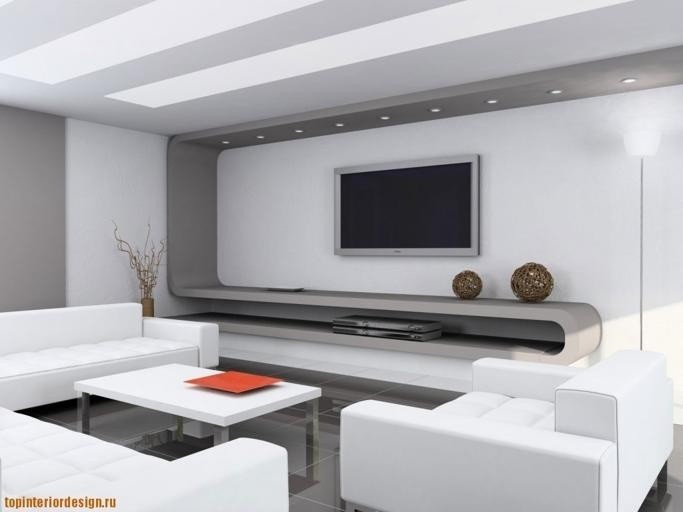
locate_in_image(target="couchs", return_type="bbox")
[0,301,221,418]
[0,408,289,512]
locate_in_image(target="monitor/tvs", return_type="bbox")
[332,153,481,256]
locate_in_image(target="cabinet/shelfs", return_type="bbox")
[161,284,602,367]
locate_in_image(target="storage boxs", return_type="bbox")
[75,361,322,481]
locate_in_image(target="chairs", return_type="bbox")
[339,354,674,512]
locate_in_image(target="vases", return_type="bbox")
[140,297,155,316]
